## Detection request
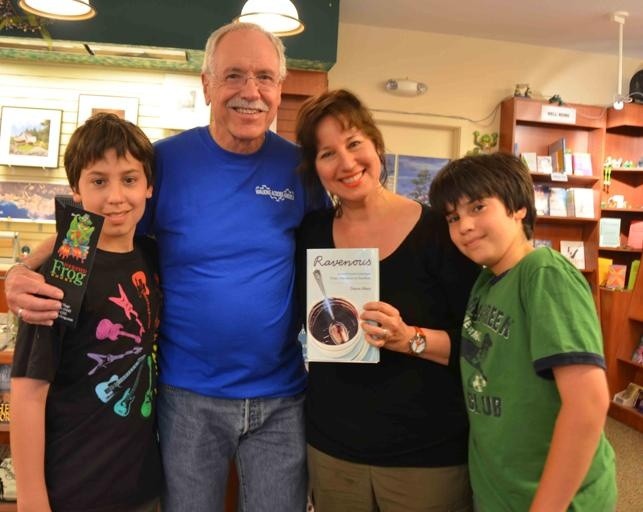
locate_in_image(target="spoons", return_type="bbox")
[312,270,348,345]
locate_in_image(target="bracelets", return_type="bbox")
[4,262,29,279]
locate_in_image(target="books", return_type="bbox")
[513,137,643,294]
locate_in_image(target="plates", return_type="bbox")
[307,305,370,363]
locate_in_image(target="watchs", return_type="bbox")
[409,326,426,358]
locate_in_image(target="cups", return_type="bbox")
[309,298,362,357]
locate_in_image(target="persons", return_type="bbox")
[9,113,163,511]
[429,150,617,512]
[295,88,483,512]
[14,245,30,260]
[4,24,336,511]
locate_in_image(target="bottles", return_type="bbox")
[6,305,19,351]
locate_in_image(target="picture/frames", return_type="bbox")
[0,105,63,170]
[75,93,140,130]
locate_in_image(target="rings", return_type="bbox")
[17,308,23,317]
[383,329,386,337]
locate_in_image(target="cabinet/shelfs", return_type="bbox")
[1,344,16,512]
[497,97,642,433]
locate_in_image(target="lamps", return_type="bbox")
[229,0,304,37]
[17,0,98,22]
[607,9,631,111]
